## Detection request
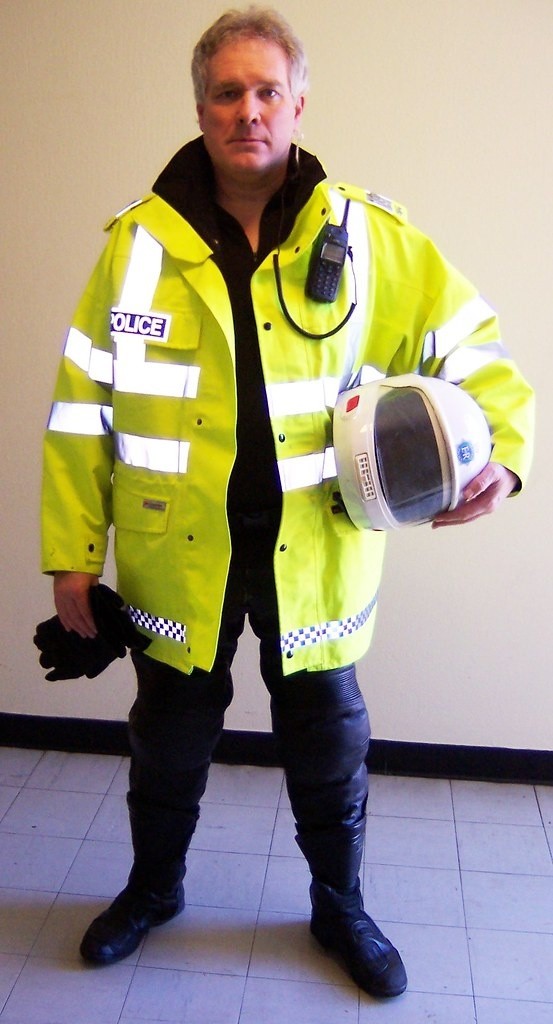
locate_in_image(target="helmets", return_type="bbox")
[333,374,492,532]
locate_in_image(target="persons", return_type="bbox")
[41,6,535,1001]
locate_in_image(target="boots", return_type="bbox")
[295,815,408,997]
[79,791,200,963]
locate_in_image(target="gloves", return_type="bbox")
[32,584,137,682]
